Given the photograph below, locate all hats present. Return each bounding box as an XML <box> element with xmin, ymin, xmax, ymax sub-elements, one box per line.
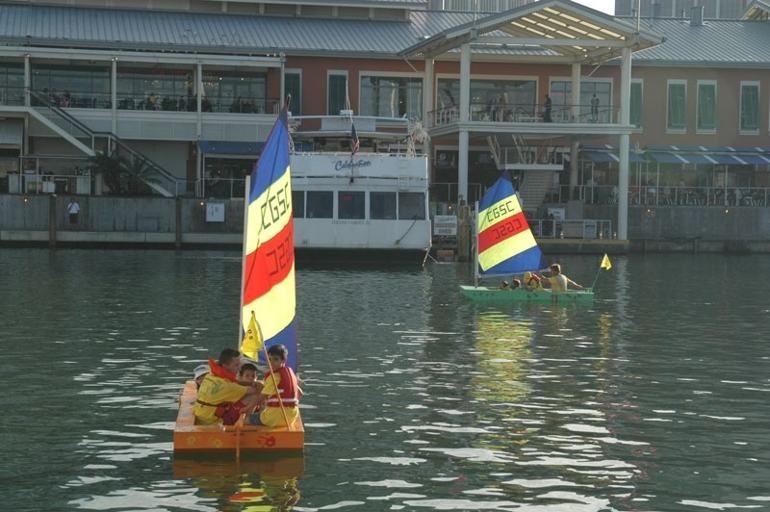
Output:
<box><xmin>193</xmin><ymin>365</ymin><xmax>212</xmax><ymax>380</ymax></box>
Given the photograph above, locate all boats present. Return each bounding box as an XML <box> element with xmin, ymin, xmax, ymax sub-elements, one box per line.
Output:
<box><xmin>283</xmin><ymin>98</ymin><xmax>436</xmax><ymax>270</ymax></box>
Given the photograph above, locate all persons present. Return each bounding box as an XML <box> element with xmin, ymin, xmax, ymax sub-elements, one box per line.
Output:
<box><xmin>29</xmin><ymin>84</ymin><xmax>268</xmax><ymax>115</ymax></box>
<box><xmin>532</xmin><ymin>262</ymin><xmax>585</xmax><ymax>293</ymax></box>
<box><xmin>67</xmin><ymin>196</ymin><xmax>81</xmax><ymax>225</ymax></box>
<box><xmin>589</xmin><ymin>93</ymin><xmax>600</xmax><ymax>122</ymax></box>
<box><xmin>193</xmin><ymin>363</ymin><xmax>210</xmax><ymax>387</ymax></box>
<box><xmin>239</xmin><ymin>362</ymin><xmax>259</xmax><ymax>383</ymax></box>
<box><xmin>585</xmin><ymin>176</ymin><xmax>770</xmax><ymax>208</ymax></box>
<box><xmin>511</xmin><ymin>278</ymin><xmax>523</xmax><ymax>289</ymax></box>
<box><xmin>544</xmin><ymin>93</ymin><xmax>552</xmax><ymax>123</ymax></box>
<box><xmin>239</xmin><ymin>343</ymin><xmax>304</xmax><ymax>428</ymax></box>
<box><xmin>500</xmin><ymin>281</ymin><xmax>509</xmax><ymax>290</ymax></box>
<box><xmin>521</xmin><ymin>271</ymin><xmax>544</xmax><ymax>291</ymax></box>
<box><xmin>192</xmin><ymin>346</ymin><xmax>266</xmax><ymax>426</ymax></box>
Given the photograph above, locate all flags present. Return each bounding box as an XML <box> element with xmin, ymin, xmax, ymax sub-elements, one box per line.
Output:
<box><xmin>599</xmin><ymin>252</ymin><xmax>612</xmax><ymax>271</ymax></box>
<box><xmin>238</xmin><ymin>312</ymin><xmax>263</xmax><ymax>364</ymax></box>
<box><xmin>350</xmin><ymin>122</ymin><xmax>360</xmax><ymax>156</ymax></box>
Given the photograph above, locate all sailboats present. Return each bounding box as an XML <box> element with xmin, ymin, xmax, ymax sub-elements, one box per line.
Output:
<box><xmin>173</xmin><ymin>93</ymin><xmax>314</xmax><ymax>460</ymax></box>
<box><xmin>450</xmin><ymin>166</ymin><xmax>615</xmax><ymax>311</ymax></box>
